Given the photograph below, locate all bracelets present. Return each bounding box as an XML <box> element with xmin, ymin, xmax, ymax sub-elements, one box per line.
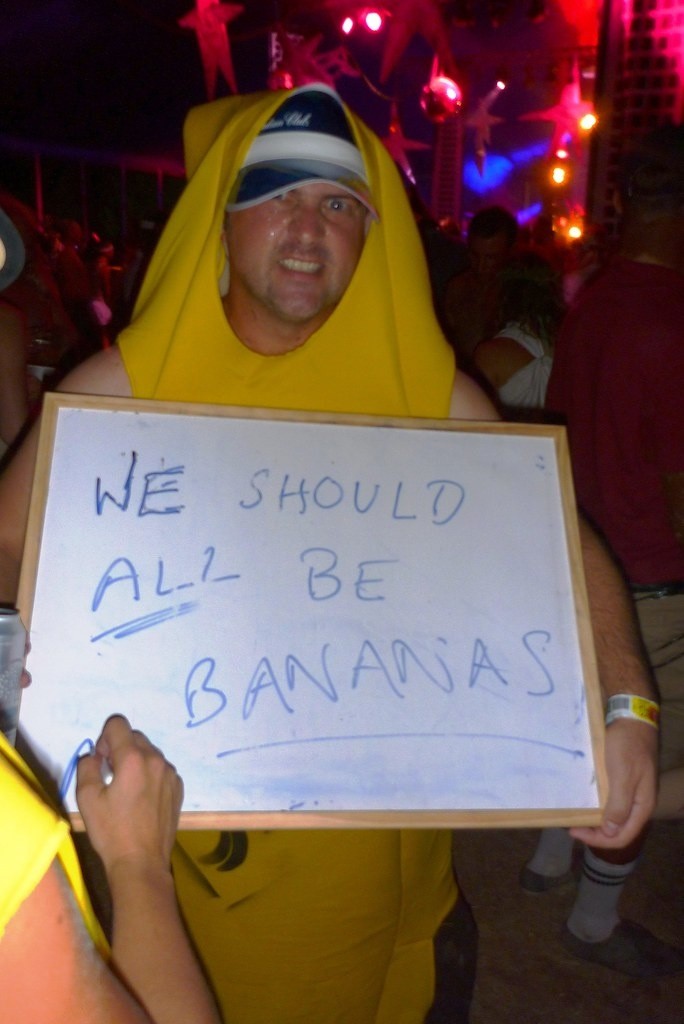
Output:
<box><xmin>604</xmin><ymin>692</ymin><xmax>661</xmax><ymax>728</ymax></box>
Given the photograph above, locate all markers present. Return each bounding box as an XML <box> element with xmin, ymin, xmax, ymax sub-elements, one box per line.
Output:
<box><xmin>89</xmin><ymin>746</ymin><xmax>114</xmax><ymax>785</ymax></box>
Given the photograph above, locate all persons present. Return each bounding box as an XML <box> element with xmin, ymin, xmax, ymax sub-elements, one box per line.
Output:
<box><xmin>1</xmin><ymin>80</ymin><xmax>684</xmax><ymax>1024</ymax></box>
<box><xmin>0</xmin><ymin>632</ymin><xmax>228</xmax><ymax>1024</ymax></box>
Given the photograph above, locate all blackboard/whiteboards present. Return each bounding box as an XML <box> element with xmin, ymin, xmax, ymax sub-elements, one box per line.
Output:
<box><xmin>15</xmin><ymin>393</ymin><xmax>608</xmax><ymax>831</ymax></box>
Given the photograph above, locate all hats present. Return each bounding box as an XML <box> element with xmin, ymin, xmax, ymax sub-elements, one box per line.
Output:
<box><xmin>223</xmin><ymin>83</ymin><xmax>385</xmax><ymax>223</ymax></box>
